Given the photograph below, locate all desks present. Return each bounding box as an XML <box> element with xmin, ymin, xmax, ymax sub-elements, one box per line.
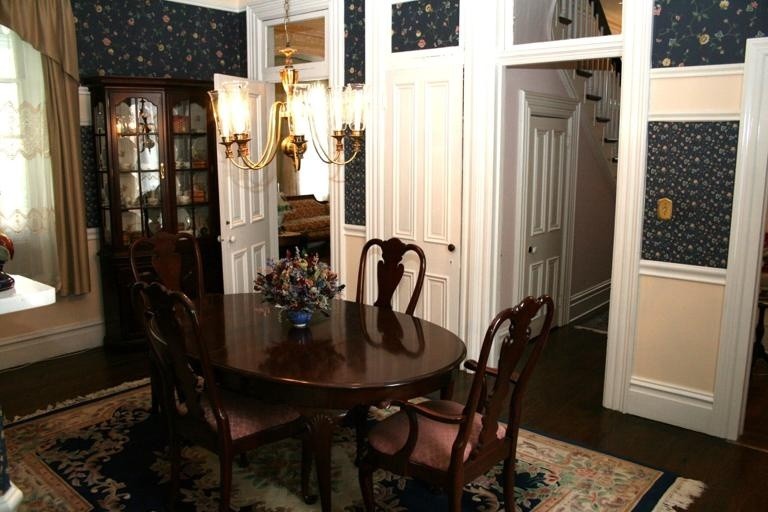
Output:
<box><xmin>279</xmin><ymin>232</ymin><xmax>301</xmax><ymax>258</ymax></box>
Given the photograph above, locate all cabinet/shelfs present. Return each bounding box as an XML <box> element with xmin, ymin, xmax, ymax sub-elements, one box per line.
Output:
<box><xmin>94</xmin><ymin>77</ymin><xmax>221</xmax><ymax>353</ymax></box>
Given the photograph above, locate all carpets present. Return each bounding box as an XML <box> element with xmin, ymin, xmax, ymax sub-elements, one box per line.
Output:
<box><xmin>1</xmin><ymin>377</ymin><xmax>707</xmax><ymax>512</ymax></box>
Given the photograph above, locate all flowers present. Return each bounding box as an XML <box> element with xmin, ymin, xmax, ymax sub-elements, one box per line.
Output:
<box><xmin>253</xmin><ymin>248</ymin><xmax>345</xmax><ymax>322</ymax></box>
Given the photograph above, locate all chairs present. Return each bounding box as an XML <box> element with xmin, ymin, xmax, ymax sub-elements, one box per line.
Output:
<box><xmin>354</xmin><ymin>238</ymin><xmax>426</xmax><ymax>316</ymax></box>
<box><xmin>359</xmin><ymin>295</ymin><xmax>554</xmax><ymax>511</ymax></box>
<box><xmin>128</xmin><ymin>231</ymin><xmax>205</xmax><ymax>298</ymax></box>
<box><xmin>132</xmin><ymin>275</ymin><xmax>335</xmax><ymax>512</ymax></box>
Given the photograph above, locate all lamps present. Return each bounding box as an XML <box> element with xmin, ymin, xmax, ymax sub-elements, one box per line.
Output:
<box><xmin>208</xmin><ymin>1</ymin><xmax>366</xmax><ymax>170</ymax></box>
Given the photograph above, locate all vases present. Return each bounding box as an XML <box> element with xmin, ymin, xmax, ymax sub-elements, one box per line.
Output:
<box><xmin>288</xmin><ymin>310</ymin><xmax>313</xmax><ymax>328</ymax></box>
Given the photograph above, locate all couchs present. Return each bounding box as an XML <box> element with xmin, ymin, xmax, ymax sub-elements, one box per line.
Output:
<box><xmin>278</xmin><ymin>195</ymin><xmax>330</xmax><ymax>239</ymax></box>
